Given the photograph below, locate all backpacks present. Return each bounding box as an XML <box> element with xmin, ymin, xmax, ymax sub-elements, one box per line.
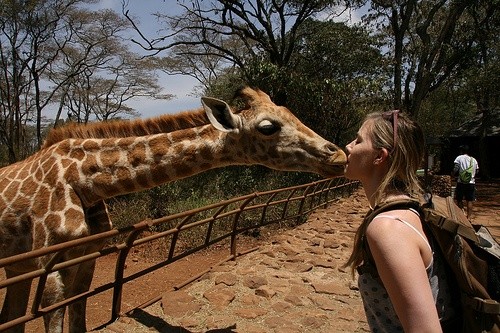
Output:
<box><xmin>356</xmin><ymin>187</ymin><xmax>499</xmax><ymax>332</ymax></box>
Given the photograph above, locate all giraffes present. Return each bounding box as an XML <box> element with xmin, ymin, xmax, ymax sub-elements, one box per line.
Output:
<box><xmin>0</xmin><ymin>86</ymin><xmax>347</xmax><ymax>333</ymax></box>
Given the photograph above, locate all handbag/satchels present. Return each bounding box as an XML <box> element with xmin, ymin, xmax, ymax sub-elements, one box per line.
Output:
<box><xmin>459</xmin><ymin>160</ymin><xmax>473</xmax><ymax>184</ymax></box>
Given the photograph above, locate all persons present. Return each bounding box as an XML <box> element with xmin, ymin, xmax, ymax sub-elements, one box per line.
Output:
<box><xmin>450</xmin><ymin>144</ymin><xmax>480</xmax><ymax>223</ymax></box>
<box><xmin>342</xmin><ymin>110</ymin><xmax>454</xmax><ymax>332</ymax></box>
